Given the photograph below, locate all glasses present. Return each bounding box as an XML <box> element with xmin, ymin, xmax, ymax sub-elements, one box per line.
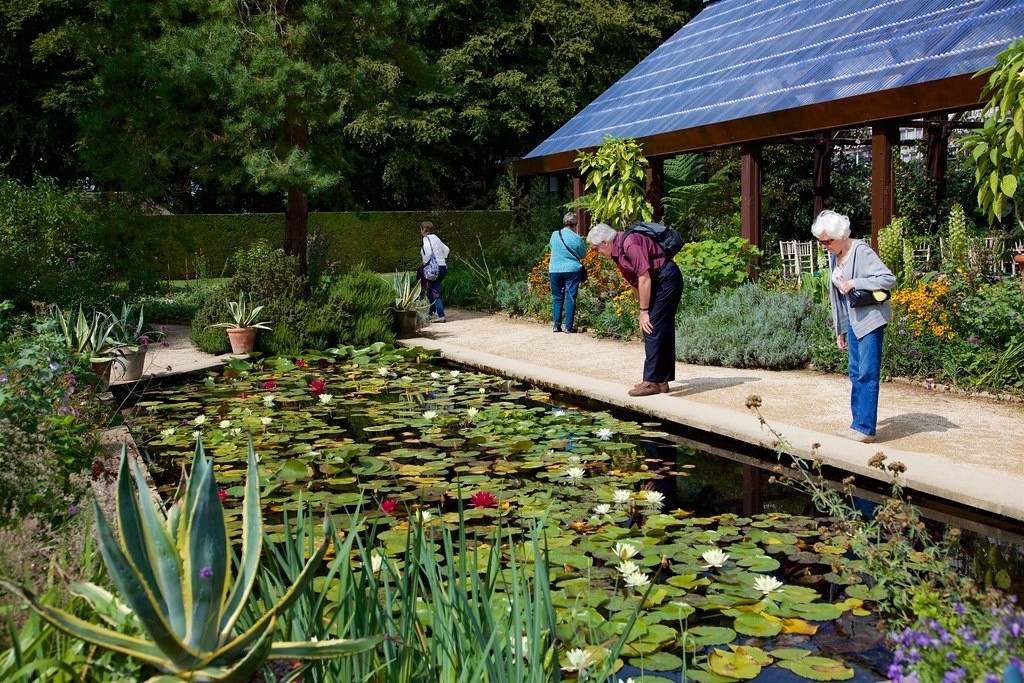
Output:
<box><xmin>819</xmin><ymin>239</ymin><xmax>835</xmax><ymax>245</ymax></box>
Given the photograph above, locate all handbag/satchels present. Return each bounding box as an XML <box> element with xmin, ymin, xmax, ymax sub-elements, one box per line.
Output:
<box><xmin>849</xmin><ymin>244</ymin><xmax>891</xmax><ymax>307</ymax></box>
<box><xmin>423</xmin><ymin>254</ymin><xmax>440</xmax><ymax>281</ymax></box>
<box><xmin>579</xmin><ymin>265</ymin><xmax>588</xmax><ymax>282</ymax></box>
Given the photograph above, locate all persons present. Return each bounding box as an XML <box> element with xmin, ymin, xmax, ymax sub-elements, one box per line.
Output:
<box><xmin>586</xmin><ymin>223</ymin><xmax>683</xmax><ymax>396</ymax></box>
<box><xmin>549</xmin><ymin>212</ymin><xmax>587</xmax><ymax>334</ymax></box>
<box><xmin>420</xmin><ymin>221</ymin><xmax>450</xmax><ymax>323</ymax></box>
<box><xmin>810</xmin><ymin>209</ymin><xmax>897</xmax><ymax>443</ymax></box>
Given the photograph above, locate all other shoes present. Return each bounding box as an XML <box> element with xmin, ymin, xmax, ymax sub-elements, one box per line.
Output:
<box><xmin>553</xmin><ymin>327</ymin><xmax>562</xmax><ymax>331</ymax></box>
<box><xmin>564</xmin><ymin>329</ymin><xmax>574</xmax><ymax>333</ymax></box>
<box><xmin>635</xmin><ymin>382</ymin><xmax>669</xmax><ymax>393</ymax></box>
<box><xmin>629</xmin><ymin>381</ymin><xmax>660</xmax><ymax>396</ymax></box>
<box><xmin>838</xmin><ymin>429</ymin><xmax>874</xmax><ymax>443</ymax></box>
<box><xmin>433</xmin><ymin>317</ymin><xmax>446</xmax><ymax>322</ymax></box>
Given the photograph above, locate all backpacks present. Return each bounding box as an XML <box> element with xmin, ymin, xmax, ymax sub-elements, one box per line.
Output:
<box><xmin>620</xmin><ymin>221</ymin><xmax>687</xmax><ymax>262</ymax></box>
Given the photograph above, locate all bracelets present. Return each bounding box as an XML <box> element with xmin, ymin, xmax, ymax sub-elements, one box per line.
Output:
<box><xmin>638</xmin><ymin>307</ymin><xmax>649</xmax><ymax>311</ymax></box>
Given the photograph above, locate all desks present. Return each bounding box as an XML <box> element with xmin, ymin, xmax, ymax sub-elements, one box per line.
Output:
<box><xmin>1014</xmin><ymin>254</ymin><xmax>1024</xmax><ymax>284</ymax></box>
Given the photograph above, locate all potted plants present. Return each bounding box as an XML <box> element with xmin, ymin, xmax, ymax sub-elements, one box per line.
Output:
<box><xmin>44</xmin><ymin>302</ymin><xmax>139</xmax><ymax>396</ymax></box>
<box><xmin>95</xmin><ymin>302</ymin><xmax>168</xmax><ymax>381</ymax></box>
<box><xmin>379</xmin><ymin>268</ymin><xmax>438</xmax><ymax>336</ymax></box>
<box><xmin>204</xmin><ymin>291</ymin><xmax>274</xmax><ymax>354</ymax></box>
